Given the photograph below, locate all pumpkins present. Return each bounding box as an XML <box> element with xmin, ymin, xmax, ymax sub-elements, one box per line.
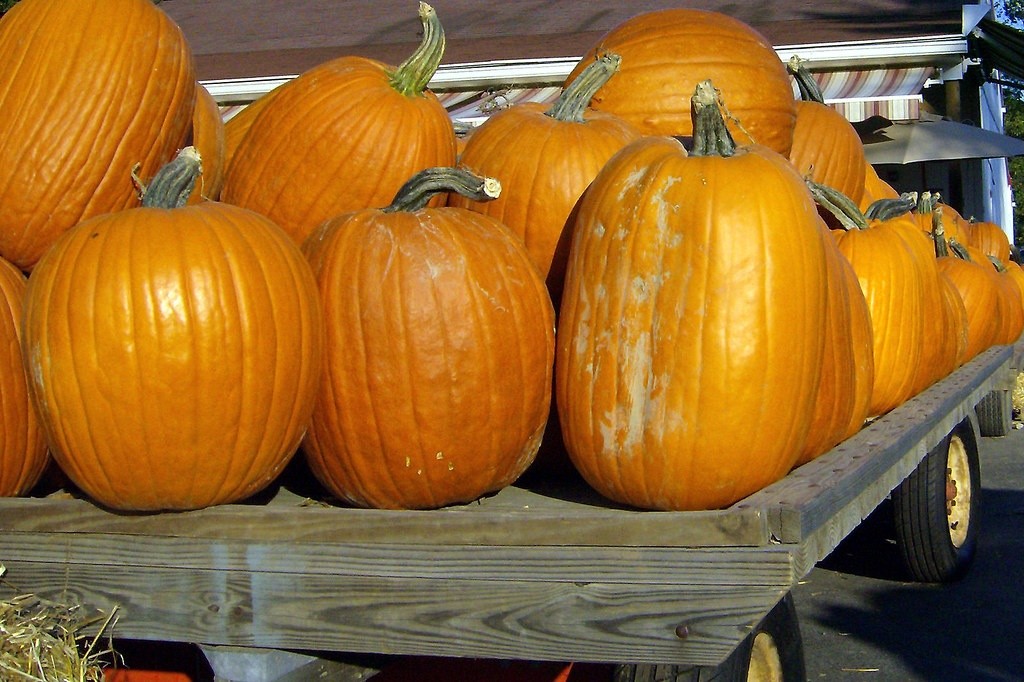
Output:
<box><xmin>0</xmin><ymin>0</ymin><xmax>1024</xmax><ymax>512</ymax></box>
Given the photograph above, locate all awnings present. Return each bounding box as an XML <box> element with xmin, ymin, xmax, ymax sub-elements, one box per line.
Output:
<box><xmin>216</xmin><ymin>62</ymin><xmax>941</xmax><ymax>128</ymax></box>
<box><xmin>967</xmin><ymin>11</ymin><xmax>1024</xmax><ymax>96</ymax></box>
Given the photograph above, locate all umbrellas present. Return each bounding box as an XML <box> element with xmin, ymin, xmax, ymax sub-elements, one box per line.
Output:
<box><xmin>860</xmin><ymin>115</ymin><xmax>1024</xmax><ymax>196</ymax></box>
